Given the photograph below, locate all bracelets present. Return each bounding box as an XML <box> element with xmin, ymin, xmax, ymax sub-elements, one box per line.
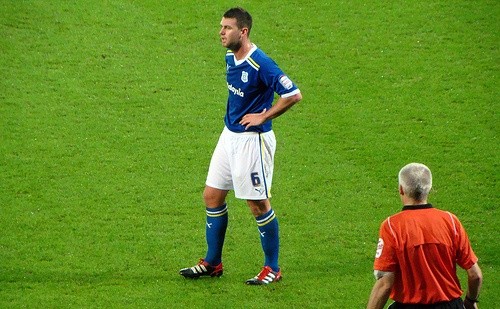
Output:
<box><xmin>465</xmin><ymin>296</ymin><xmax>479</xmax><ymax>303</ymax></box>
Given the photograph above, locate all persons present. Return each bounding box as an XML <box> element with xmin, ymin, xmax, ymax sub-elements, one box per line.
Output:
<box><xmin>365</xmin><ymin>163</ymin><xmax>482</xmax><ymax>309</ymax></box>
<box><xmin>179</xmin><ymin>7</ymin><xmax>303</xmax><ymax>285</ymax></box>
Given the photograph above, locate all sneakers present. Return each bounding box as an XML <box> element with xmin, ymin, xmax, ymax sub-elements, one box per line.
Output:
<box><xmin>245</xmin><ymin>265</ymin><xmax>282</xmax><ymax>286</ymax></box>
<box><xmin>179</xmin><ymin>258</ymin><xmax>223</xmax><ymax>280</ymax></box>
